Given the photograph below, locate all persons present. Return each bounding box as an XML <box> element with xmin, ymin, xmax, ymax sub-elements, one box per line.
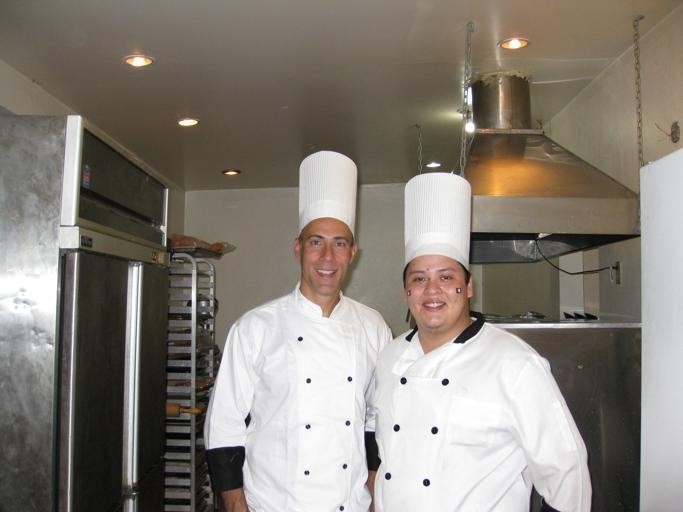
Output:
<box><xmin>361</xmin><ymin>244</ymin><xmax>591</xmax><ymax>512</ymax></box>
<box><xmin>204</xmin><ymin>218</ymin><xmax>397</xmax><ymax>512</ymax></box>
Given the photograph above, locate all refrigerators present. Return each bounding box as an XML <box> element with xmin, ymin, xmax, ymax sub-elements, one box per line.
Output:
<box><xmin>0</xmin><ymin>113</ymin><xmax>170</xmax><ymax>510</ymax></box>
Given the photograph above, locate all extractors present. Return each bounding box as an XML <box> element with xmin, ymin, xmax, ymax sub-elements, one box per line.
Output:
<box><xmin>457</xmin><ymin>75</ymin><xmax>640</xmax><ymax>263</ymax></box>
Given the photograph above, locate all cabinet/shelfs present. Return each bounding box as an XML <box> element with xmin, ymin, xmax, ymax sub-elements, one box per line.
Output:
<box><xmin>164</xmin><ymin>245</ymin><xmax>219</xmax><ymax>512</ymax></box>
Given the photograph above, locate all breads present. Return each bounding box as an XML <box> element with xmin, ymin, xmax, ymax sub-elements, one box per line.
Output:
<box><xmin>171</xmin><ymin>232</ymin><xmax>225</xmax><ymax>254</ymax></box>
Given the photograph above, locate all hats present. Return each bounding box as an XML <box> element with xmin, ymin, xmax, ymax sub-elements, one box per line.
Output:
<box><xmin>298</xmin><ymin>150</ymin><xmax>358</xmax><ymax>240</ymax></box>
<box><xmin>404</xmin><ymin>172</ymin><xmax>472</xmax><ymax>273</ymax></box>
